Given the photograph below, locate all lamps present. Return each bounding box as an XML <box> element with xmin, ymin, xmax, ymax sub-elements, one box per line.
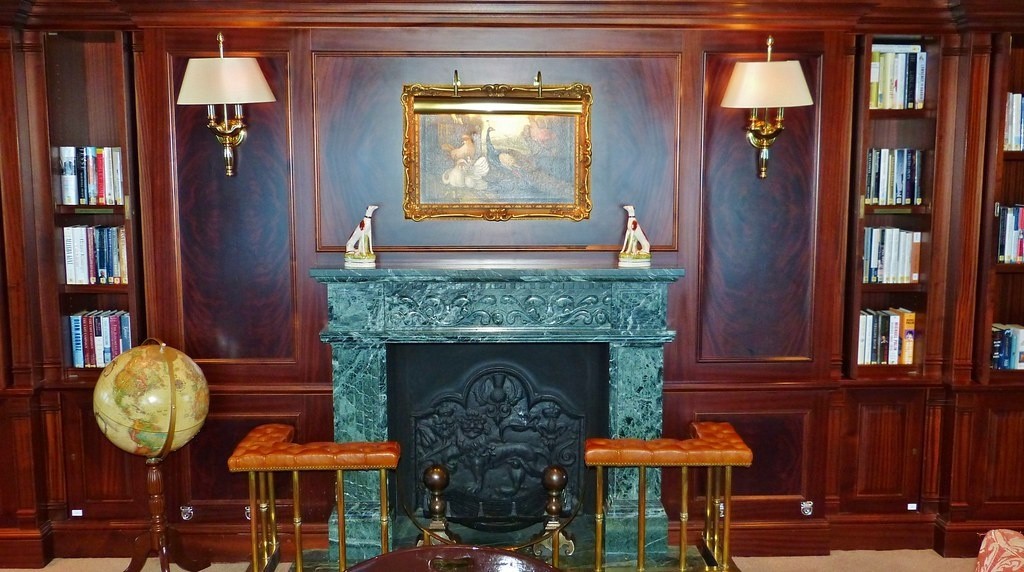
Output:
<box><xmin>721</xmin><ymin>35</ymin><xmax>814</xmax><ymax>181</ymax></box>
<box><xmin>179</xmin><ymin>33</ymin><xmax>276</xmax><ymax>179</ymax></box>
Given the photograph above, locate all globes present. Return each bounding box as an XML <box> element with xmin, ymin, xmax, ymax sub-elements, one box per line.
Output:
<box><xmin>94</xmin><ymin>338</ymin><xmax>211</xmax><ymax>572</ymax></box>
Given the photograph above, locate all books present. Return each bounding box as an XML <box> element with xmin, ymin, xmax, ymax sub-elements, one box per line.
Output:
<box><xmin>64</xmin><ymin>225</ymin><xmax>128</xmax><ymax>285</ymax></box>
<box><xmin>869</xmin><ymin>44</ymin><xmax>926</xmax><ymax>110</ymax></box>
<box><xmin>1003</xmin><ymin>92</ymin><xmax>1024</xmax><ymax>152</ymax></box>
<box><xmin>858</xmin><ymin>307</ymin><xmax>915</xmax><ymax>365</ymax></box>
<box><xmin>69</xmin><ymin>309</ymin><xmax>131</xmax><ymax>369</ymax></box>
<box><xmin>991</xmin><ymin>324</ymin><xmax>1024</xmax><ymax>369</ymax></box>
<box><xmin>59</xmin><ymin>147</ymin><xmax>125</xmax><ymax>206</ymax></box>
<box><xmin>997</xmin><ymin>204</ymin><xmax>1024</xmax><ymax>264</ymax></box>
<box><xmin>865</xmin><ymin>147</ymin><xmax>923</xmax><ymax>206</ymax></box>
<box><xmin>862</xmin><ymin>226</ymin><xmax>922</xmax><ymax>283</ymax></box>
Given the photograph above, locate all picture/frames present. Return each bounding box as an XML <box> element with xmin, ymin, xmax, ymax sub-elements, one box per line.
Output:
<box><xmin>402</xmin><ymin>84</ymin><xmax>592</xmax><ymax>223</ymax></box>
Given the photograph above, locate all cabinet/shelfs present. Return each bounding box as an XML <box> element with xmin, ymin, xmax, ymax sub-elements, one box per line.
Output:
<box><xmin>0</xmin><ymin>22</ymin><xmax>153</xmax><ymax>520</ymax></box>
<box><xmin>975</xmin><ymin>26</ymin><xmax>1024</xmax><ymax>385</ymax></box>
<box><xmin>850</xmin><ymin>30</ymin><xmax>965</xmax><ymax>380</ymax></box>
